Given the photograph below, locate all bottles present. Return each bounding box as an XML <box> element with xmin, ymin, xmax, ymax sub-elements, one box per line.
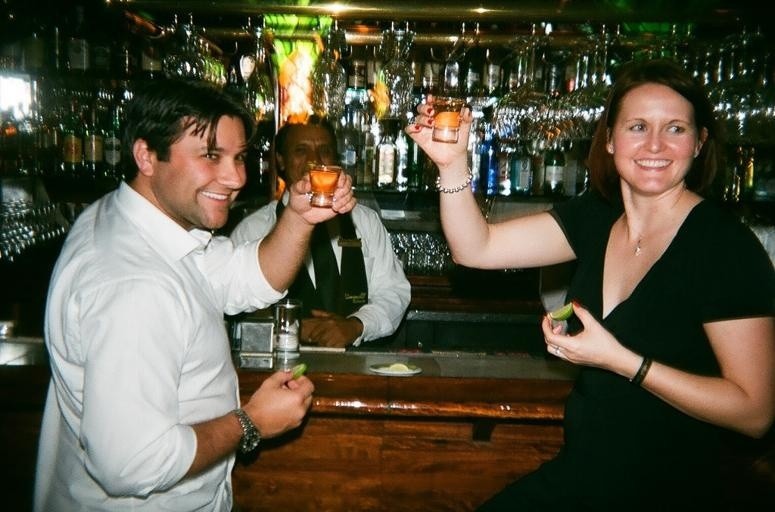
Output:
<box><xmin>723</xmin><ymin>129</ymin><xmax>755</xmax><ymax>203</ymax></box>
<box><xmin>345</xmin><ymin>20</ymin><xmax>775</xmax><ymax>94</ymax></box>
<box><xmin>225</xmin><ymin>39</ymin><xmax>244</xmax><ymax>88</ymax></box>
<box><xmin>342</xmin><ymin>104</ymin><xmax>435</xmax><ymax>194</ymax></box>
<box><xmin>0</xmin><ymin>1</ymin><xmax>160</xmax><ymax>179</ymax></box>
<box><xmin>470</xmin><ymin>99</ymin><xmax>588</xmax><ymax>198</ymax></box>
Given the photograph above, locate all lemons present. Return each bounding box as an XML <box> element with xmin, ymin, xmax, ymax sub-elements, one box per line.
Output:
<box><xmin>289</xmin><ymin>364</ymin><xmax>308</xmax><ymax>378</ymax></box>
<box><xmin>371</xmin><ymin>361</ymin><xmax>416</xmax><ymax>372</ymax></box>
<box><xmin>549</xmin><ymin>303</ymin><xmax>572</xmax><ymax>321</ymax></box>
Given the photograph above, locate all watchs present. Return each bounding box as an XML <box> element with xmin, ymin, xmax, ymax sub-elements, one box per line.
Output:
<box><xmin>232</xmin><ymin>409</ymin><xmax>261</xmax><ymax>454</ymax></box>
<box><xmin>628</xmin><ymin>355</ymin><xmax>653</xmax><ymax>385</ymax></box>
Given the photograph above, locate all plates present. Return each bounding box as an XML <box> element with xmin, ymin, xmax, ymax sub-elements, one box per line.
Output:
<box><xmin>370</xmin><ymin>364</ymin><xmax>422</xmax><ymax>378</ymax></box>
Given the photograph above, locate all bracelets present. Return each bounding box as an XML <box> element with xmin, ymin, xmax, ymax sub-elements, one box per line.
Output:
<box><xmin>436</xmin><ymin>168</ymin><xmax>473</xmax><ymax>193</ymax></box>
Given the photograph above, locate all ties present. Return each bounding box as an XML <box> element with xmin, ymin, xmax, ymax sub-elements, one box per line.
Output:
<box><xmin>312</xmin><ymin>221</ymin><xmax>341</xmax><ymax>314</ymax></box>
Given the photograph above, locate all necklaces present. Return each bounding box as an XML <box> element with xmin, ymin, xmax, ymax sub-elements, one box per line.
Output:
<box><xmin>635</xmin><ymin>234</ymin><xmax>645</xmax><ymax>256</ymax></box>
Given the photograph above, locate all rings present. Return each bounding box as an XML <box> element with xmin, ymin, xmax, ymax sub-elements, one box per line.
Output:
<box><xmin>556</xmin><ymin>349</ymin><xmax>559</xmax><ymax>354</ymax></box>
<box><xmin>556</xmin><ymin>346</ymin><xmax>561</xmax><ymax>350</ymax></box>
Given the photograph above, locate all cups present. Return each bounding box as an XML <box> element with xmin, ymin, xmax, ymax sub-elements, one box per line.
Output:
<box><xmin>272</xmin><ymin>298</ymin><xmax>302</xmax><ymax>352</ymax></box>
<box><xmin>389</xmin><ymin>227</ymin><xmax>451</xmax><ymax>275</ymax></box>
<box><xmin>433</xmin><ymin>93</ymin><xmax>463</xmax><ymax>144</ymax></box>
<box><xmin>273</xmin><ymin>353</ymin><xmax>301</xmax><ymax>373</ymax></box>
<box><xmin>308</xmin><ymin>161</ymin><xmax>341</xmax><ymax>209</ymax></box>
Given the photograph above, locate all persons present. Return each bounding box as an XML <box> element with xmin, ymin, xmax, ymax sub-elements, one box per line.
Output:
<box><xmin>228</xmin><ymin>116</ymin><xmax>411</xmax><ymax>349</ymax></box>
<box><xmin>35</xmin><ymin>80</ymin><xmax>356</xmax><ymax>512</ymax></box>
<box><xmin>406</xmin><ymin>59</ymin><xmax>775</xmax><ymax>512</ymax></box>
